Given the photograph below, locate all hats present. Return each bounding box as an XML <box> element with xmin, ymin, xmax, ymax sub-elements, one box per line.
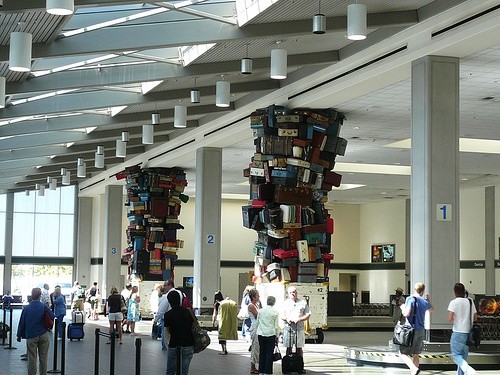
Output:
<box><xmin>395</xmin><ymin>288</ymin><xmax>403</xmax><ymax>293</ymax></box>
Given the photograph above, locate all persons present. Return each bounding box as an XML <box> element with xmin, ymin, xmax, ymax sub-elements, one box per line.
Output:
<box><xmin>447</xmin><ymin>283</ymin><xmax>478</xmax><ymax>375</ymax></box>
<box><xmin>248</xmin><ymin>288</ymin><xmax>260</xmax><ymax>375</ymax></box>
<box><xmin>41</xmin><ymin>284</ymin><xmax>50</xmax><ymax>309</ymax></box>
<box><xmin>16</xmin><ymin>288</ymin><xmax>55</xmax><ymax>375</ymax></box>
<box><xmin>70</xmin><ymin>281</ymin><xmax>89</xmax><ymax>321</ymax></box>
<box><xmin>280</xmin><ymin>286</ymin><xmax>311</xmax><ymax>373</ymax></box>
<box><xmin>164</xmin><ymin>291</ymin><xmax>198</xmax><ymax>375</ymax></box>
<box><xmin>52</xmin><ymin>287</ymin><xmax>66</xmax><ymax>341</ymax></box>
<box><xmin>106</xmin><ymin>285</ymin><xmax>126</xmax><ymax>345</ymax></box>
<box><xmin>242</xmin><ymin>286</ymin><xmax>255</xmax><ymax>337</ymax></box>
<box><xmin>2</xmin><ymin>290</ymin><xmax>14</xmax><ymax>310</ymax></box>
<box><xmin>150</xmin><ymin>284</ymin><xmax>161</xmax><ymax>324</ymax></box>
<box><xmin>254</xmin><ymin>296</ymin><xmax>279</xmax><ymax>375</ymax></box>
<box><xmin>128</xmin><ymin>285</ymin><xmax>140</xmax><ymax>336</ymax></box>
<box><xmin>47</xmin><ymin>285</ymin><xmax>66</xmax><ymax>332</ymax></box>
<box><xmin>154</xmin><ymin>279</ymin><xmax>185</xmax><ymax>350</ymax></box>
<box><xmin>120</xmin><ymin>283</ymin><xmax>132</xmax><ymax>334</ymax></box>
<box><xmin>399</xmin><ymin>282</ymin><xmax>433</xmax><ymax>375</ymax></box>
<box><xmin>390</xmin><ymin>287</ymin><xmax>406</xmax><ymax>342</ymax></box>
<box><xmin>213</xmin><ymin>291</ymin><xmax>227</xmax><ymax>355</ymax></box>
<box><xmin>85</xmin><ymin>282</ymin><xmax>101</xmax><ymax>320</ymax></box>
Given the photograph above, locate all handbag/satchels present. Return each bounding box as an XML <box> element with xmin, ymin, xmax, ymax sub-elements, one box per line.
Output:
<box><xmin>187</xmin><ymin>306</ymin><xmax>210</xmax><ymax>354</ymax></box>
<box><xmin>120</xmin><ymin>295</ymin><xmax>128</xmax><ymax>313</ymax></box>
<box><xmin>466</xmin><ymin>299</ymin><xmax>483</xmax><ymax>347</ymax></box>
<box><xmin>237</xmin><ymin>296</ymin><xmax>249</xmax><ymax>320</ymax></box>
<box><xmin>182</xmin><ymin>292</ymin><xmax>194</xmax><ymax>314</ymax></box>
<box><xmin>44</xmin><ymin>308</ymin><xmax>53</xmax><ymax>329</ymax></box>
<box><xmin>272</xmin><ymin>345</ymin><xmax>281</xmax><ymax>361</ymax></box>
<box><xmin>393</xmin><ymin>297</ymin><xmax>416</xmax><ymax>347</ymax></box>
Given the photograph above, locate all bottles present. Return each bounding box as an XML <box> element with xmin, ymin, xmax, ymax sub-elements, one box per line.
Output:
<box><xmin>389</xmin><ymin>338</ymin><xmax>393</xmax><ymax>348</ymax></box>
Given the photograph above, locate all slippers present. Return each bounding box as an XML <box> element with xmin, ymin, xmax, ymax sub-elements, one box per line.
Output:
<box><xmin>250</xmin><ymin>370</ymin><xmax>258</xmax><ymax>373</ymax></box>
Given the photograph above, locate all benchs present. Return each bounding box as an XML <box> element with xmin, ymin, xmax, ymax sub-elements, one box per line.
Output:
<box><xmin>27</xmin><ymin>296</ymin><xmax>49</xmax><ymax>307</ymax></box>
<box><xmin>0</xmin><ymin>295</ymin><xmax>23</xmax><ymax>309</ymax></box>
<box><xmin>61</xmin><ymin>294</ymin><xmax>72</xmax><ymax>308</ymax></box>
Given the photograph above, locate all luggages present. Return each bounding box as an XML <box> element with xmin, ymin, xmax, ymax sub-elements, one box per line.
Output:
<box><xmin>72</xmin><ymin>306</ymin><xmax>85</xmax><ymax>323</ymax></box>
<box><xmin>68</xmin><ymin>311</ymin><xmax>85</xmax><ymax>342</ymax></box>
<box><xmin>241</xmin><ymin>104</ymin><xmax>348</xmax><ymax>284</ymax></box>
<box><xmin>124</xmin><ymin>165</ymin><xmax>189</xmax><ymax>281</ymax></box>
<box><xmin>282</xmin><ymin>321</ymin><xmax>304</xmax><ymax>374</ymax></box>
